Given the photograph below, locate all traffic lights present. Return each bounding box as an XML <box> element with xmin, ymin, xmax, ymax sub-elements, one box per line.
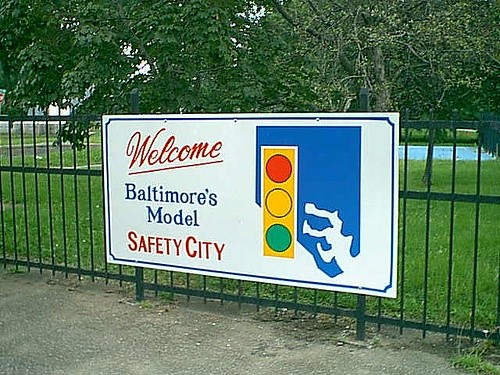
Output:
<box><xmin>261</xmin><ymin>145</ymin><xmax>297</xmax><ymax>261</ymax></box>
